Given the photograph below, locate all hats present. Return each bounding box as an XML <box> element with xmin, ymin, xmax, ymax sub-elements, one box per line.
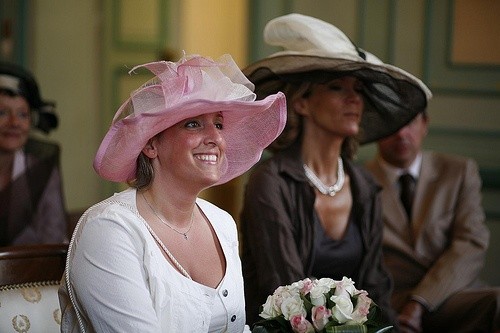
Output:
<box><xmin>240</xmin><ymin>11</ymin><xmax>433</xmax><ymax>152</ymax></box>
<box><xmin>92</xmin><ymin>50</ymin><xmax>287</xmax><ymax>186</ymax></box>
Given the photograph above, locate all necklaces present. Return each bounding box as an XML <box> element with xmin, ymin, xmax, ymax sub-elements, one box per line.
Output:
<box><xmin>300</xmin><ymin>156</ymin><xmax>346</xmax><ymax>197</ymax></box>
<box><xmin>140</xmin><ymin>188</ymin><xmax>195</xmax><ymax>239</ymax></box>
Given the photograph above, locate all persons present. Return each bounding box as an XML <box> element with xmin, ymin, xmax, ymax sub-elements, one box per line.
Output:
<box><xmin>361</xmin><ymin>104</ymin><xmax>490</xmax><ymax>333</ymax></box>
<box><xmin>58</xmin><ymin>51</ymin><xmax>287</xmax><ymax>333</ymax></box>
<box><xmin>238</xmin><ymin>13</ymin><xmax>432</xmax><ymax>333</ymax></box>
<box><xmin>0</xmin><ymin>16</ymin><xmax>60</xmax><ymax>136</ymax></box>
<box><xmin>0</xmin><ymin>92</ymin><xmax>68</xmax><ymax>245</ymax></box>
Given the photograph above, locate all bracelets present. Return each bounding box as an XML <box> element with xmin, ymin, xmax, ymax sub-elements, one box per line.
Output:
<box><xmin>408</xmin><ymin>293</ymin><xmax>431</xmax><ymax>312</ymax></box>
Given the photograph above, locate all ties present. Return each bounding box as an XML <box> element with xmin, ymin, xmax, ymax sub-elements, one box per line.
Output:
<box><xmin>397</xmin><ymin>174</ymin><xmax>415</xmax><ymax>225</ymax></box>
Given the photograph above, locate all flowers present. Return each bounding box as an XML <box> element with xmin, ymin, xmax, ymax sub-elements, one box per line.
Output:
<box><xmin>253</xmin><ymin>276</ymin><xmax>399</xmax><ymax>333</ymax></box>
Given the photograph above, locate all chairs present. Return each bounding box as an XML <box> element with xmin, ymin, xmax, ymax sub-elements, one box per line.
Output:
<box><xmin>0</xmin><ymin>243</ymin><xmax>69</xmax><ymax>333</ymax></box>
